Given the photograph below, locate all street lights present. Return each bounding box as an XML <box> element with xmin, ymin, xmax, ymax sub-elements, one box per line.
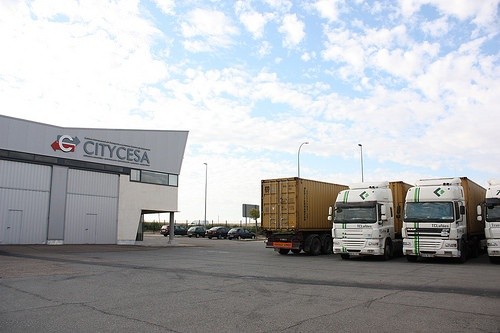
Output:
<box><xmin>203</xmin><ymin>163</ymin><xmax>207</xmax><ymax>225</ymax></box>
<box><xmin>358</xmin><ymin>144</ymin><xmax>363</xmax><ymax>182</ymax></box>
<box><xmin>298</xmin><ymin>142</ymin><xmax>309</xmax><ymax>177</ymax></box>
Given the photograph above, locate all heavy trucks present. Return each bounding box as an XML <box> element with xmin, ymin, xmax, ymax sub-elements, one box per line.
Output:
<box><xmin>328</xmin><ymin>181</ymin><xmax>414</xmax><ymax>261</ymax></box>
<box><xmin>260</xmin><ymin>177</ymin><xmax>350</xmax><ymax>257</ymax></box>
<box><xmin>397</xmin><ymin>177</ymin><xmax>487</xmax><ymax>261</ymax></box>
<box><xmin>477</xmin><ymin>179</ymin><xmax>500</xmax><ymax>263</ymax></box>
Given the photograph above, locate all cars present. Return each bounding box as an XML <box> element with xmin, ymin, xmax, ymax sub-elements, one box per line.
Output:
<box><xmin>187</xmin><ymin>226</ymin><xmax>206</xmax><ymax>238</ymax></box>
<box><xmin>206</xmin><ymin>227</ymin><xmax>227</xmax><ymax>239</ymax></box>
<box><xmin>160</xmin><ymin>225</ymin><xmax>187</xmax><ymax>237</ymax></box>
<box><xmin>227</xmin><ymin>227</ymin><xmax>256</xmax><ymax>240</ymax></box>
